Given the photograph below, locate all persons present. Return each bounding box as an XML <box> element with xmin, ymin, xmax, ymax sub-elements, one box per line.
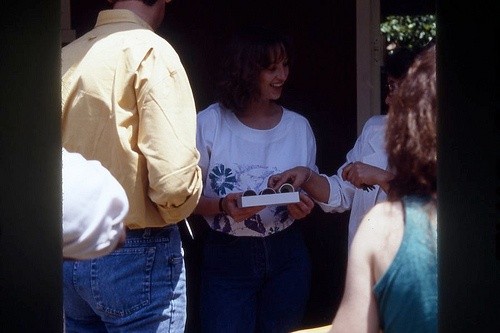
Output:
<box><xmin>191</xmin><ymin>20</ymin><xmax>318</xmax><ymax>333</ymax></box>
<box><xmin>329</xmin><ymin>44</ymin><xmax>438</xmax><ymax>333</ymax></box>
<box><xmin>267</xmin><ymin>44</ymin><xmax>429</xmax><ymax>259</ymax></box>
<box><xmin>60</xmin><ymin>0</ymin><xmax>203</xmax><ymax>333</ymax></box>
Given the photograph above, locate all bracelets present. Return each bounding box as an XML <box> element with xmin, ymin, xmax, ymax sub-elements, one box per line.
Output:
<box><xmin>305</xmin><ymin>168</ymin><xmax>313</xmax><ymax>183</ymax></box>
<box><xmin>219</xmin><ymin>197</ymin><xmax>225</xmax><ymax>214</ymax></box>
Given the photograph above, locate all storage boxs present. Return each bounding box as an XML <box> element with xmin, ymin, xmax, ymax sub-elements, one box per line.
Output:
<box><xmin>237</xmin><ymin>191</ymin><xmax>300</xmax><ymax>208</ymax></box>
